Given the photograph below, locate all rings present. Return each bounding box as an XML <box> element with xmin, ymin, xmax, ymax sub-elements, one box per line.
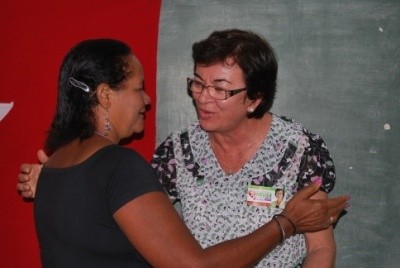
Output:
<box><xmin>331</xmin><ymin>216</ymin><xmax>334</xmax><ymax>224</ymax></box>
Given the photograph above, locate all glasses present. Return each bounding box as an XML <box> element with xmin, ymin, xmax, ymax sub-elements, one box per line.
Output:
<box><xmin>187</xmin><ymin>77</ymin><xmax>249</xmax><ymax>100</ymax></box>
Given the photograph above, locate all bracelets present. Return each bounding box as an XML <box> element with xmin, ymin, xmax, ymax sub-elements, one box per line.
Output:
<box><xmin>272</xmin><ymin>214</ymin><xmax>296</xmax><ymax>243</ymax></box>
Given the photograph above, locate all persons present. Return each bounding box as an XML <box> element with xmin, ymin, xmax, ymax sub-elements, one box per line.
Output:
<box><xmin>272</xmin><ymin>189</ymin><xmax>286</xmax><ymax>209</ymax></box>
<box><xmin>17</xmin><ymin>29</ymin><xmax>337</xmax><ymax>268</ymax></box>
<box><xmin>32</xmin><ymin>37</ymin><xmax>351</xmax><ymax>268</ymax></box>
